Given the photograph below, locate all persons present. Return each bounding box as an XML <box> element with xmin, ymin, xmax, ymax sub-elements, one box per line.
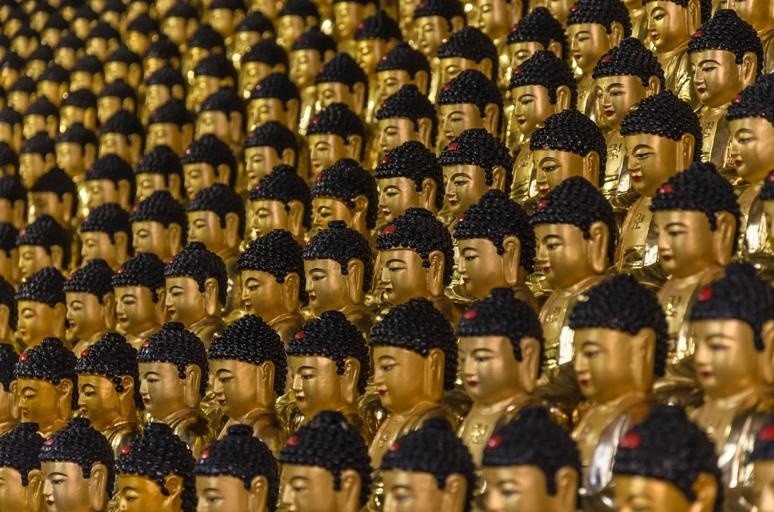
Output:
<box><xmin>2</xmin><ymin>2</ymin><xmax>772</xmax><ymax>512</ymax></box>
